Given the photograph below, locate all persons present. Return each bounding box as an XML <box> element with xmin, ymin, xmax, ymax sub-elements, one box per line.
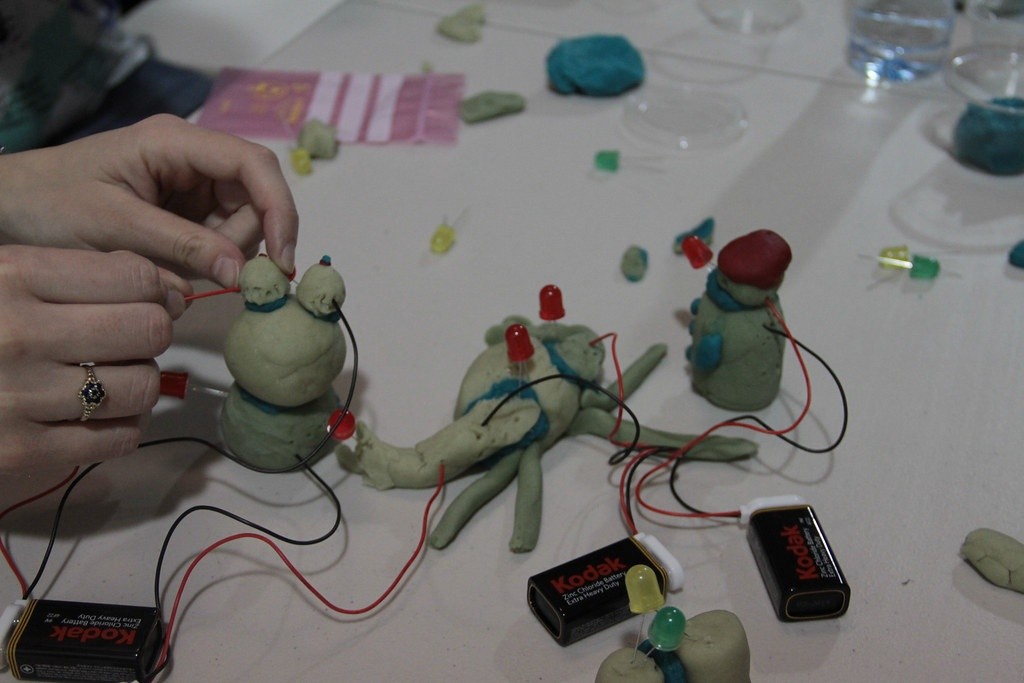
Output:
<box><xmin>0</xmin><ymin>113</ymin><xmax>299</xmax><ymax>473</ymax></box>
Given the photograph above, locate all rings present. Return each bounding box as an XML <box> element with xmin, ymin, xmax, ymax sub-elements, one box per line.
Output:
<box><xmin>76</xmin><ymin>362</ymin><xmax>106</xmax><ymax>424</ymax></box>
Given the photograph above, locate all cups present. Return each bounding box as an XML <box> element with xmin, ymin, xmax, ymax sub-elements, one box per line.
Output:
<box><xmin>945</xmin><ymin>0</ymin><xmax>1024</xmax><ymax>176</ymax></box>
<box><xmin>696</xmin><ymin>0</ymin><xmax>804</xmax><ymax>34</ymax></box>
<box><xmin>842</xmin><ymin>0</ymin><xmax>955</xmax><ymax>82</ymax></box>
